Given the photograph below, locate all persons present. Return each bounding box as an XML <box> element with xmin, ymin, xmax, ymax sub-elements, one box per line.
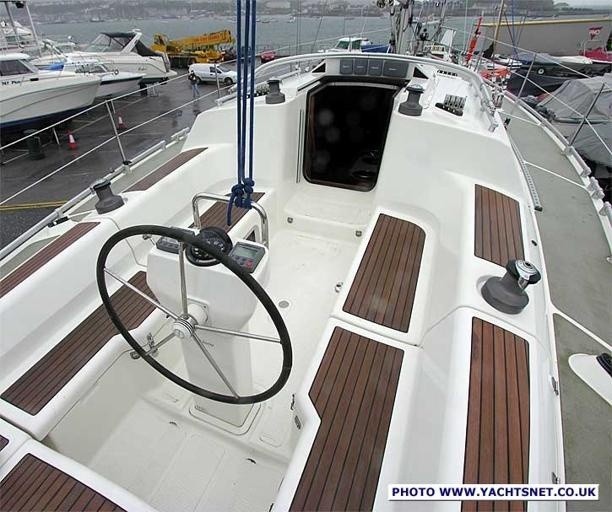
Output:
<box><xmin>187</xmin><ymin>70</ymin><xmax>202</xmax><ymax>99</ymax></box>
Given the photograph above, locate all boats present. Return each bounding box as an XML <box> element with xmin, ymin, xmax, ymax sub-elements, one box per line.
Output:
<box><xmin>0</xmin><ymin>0</ymin><xmax>612</xmax><ymax>511</ymax></box>
